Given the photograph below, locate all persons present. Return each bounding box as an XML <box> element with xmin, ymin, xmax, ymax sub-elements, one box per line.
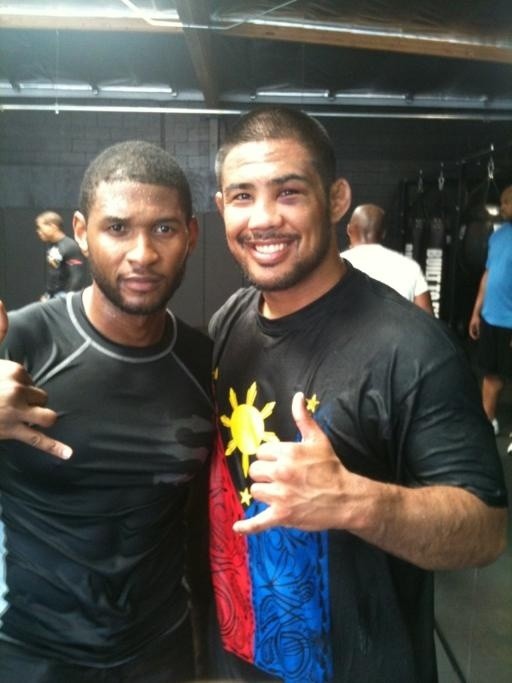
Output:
<box><xmin>209</xmin><ymin>103</ymin><xmax>512</xmax><ymax>682</ymax></box>
<box><xmin>340</xmin><ymin>202</ymin><xmax>433</xmax><ymax>317</ymax></box>
<box><xmin>33</xmin><ymin>210</ymin><xmax>85</xmax><ymax>300</ymax></box>
<box><xmin>0</xmin><ymin>138</ymin><xmax>215</xmax><ymax>683</ymax></box>
<box><xmin>468</xmin><ymin>187</ymin><xmax>512</xmax><ymax>455</ymax></box>
